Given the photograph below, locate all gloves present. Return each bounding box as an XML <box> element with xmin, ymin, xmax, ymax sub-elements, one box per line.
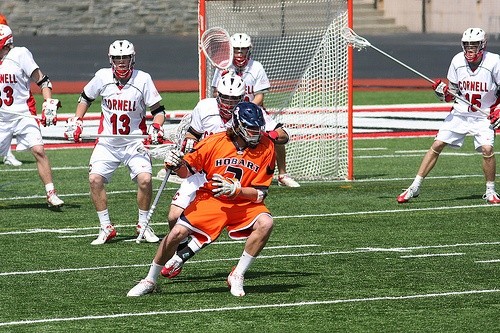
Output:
<box><xmin>149</xmin><ymin>123</ymin><xmax>164</xmax><ymax>145</ymax></box>
<box><xmin>63</xmin><ymin>117</ymin><xmax>83</xmax><ymax>143</ymax></box>
<box><xmin>211</xmin><ymin>173</ymin><xmax>242</xmax><ymax>200</ymax></box>
<box><xmin>487</xmin><ymin>104</ymin><xmax>500</xmax><ymax>129</ymax></box>
<box><xmin>431</xmin><ymin>79</ymin><xmax>449</xmax><ymax>101</ymax></box>
<box><xmin>181</xmin><ymin>137</ymin><xmax>198</xmax><ymax>155</ymax></box>
<box><xmin>42</xmin><ymin>98</ymin><xmax>62</xmax><ymax>127</ymax></box>
<box><xmin>164</xmin><ymin>149</ymin><xmax>184</xmax><ymax>172</ymax></box>
<box><xmin>266</xmin><ymin>130</ymin><xmax>279</xmax><ymax>142</ymax></box>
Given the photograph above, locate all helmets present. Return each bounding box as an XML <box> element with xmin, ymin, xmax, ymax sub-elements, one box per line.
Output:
<box><xmin>0</xmin><ymin>24</ymin><xmax>13</xmax><ymax>51</ymax></box>
<box><xmin>233</xmin><ymin>102</ymin><xmax>266</xmax><ymax>148</ymax></box>
<box><xmin>108</xmin><ymin>40</ymin><xmax>136</xmax><ymax>79</ymax></box>
<box><xmin>218</xmin><ymin>74</ymin><xmax>246</xmax><ymax>119</ymax></box>
<box><xmin>460</xmin><ymin>27</ymin><xmax>487</xmax><ymax>63</ymax></box>
<box><xmin>231</xmin><ymin>32</ymin><xmax>251</xmax><ymax>67</ymax></box>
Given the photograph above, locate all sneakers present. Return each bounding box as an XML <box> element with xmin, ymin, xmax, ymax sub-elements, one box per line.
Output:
<box><xmin>48</xmin><ymin>195</ymin><xmax>64</xmax><ymax>208</ymax></box>
<box><xmin>90</xmin><ymin>225</ymin><xmax>116</xmax><ymax>245</ymax></box>
<box><xmin>278</xmin><ymin>174</ymin><xmax>300</xmax><ymax>187</ymax></box>
<box><xmin>127</xmin><ymin>279</ymin><xmax>159</xmax><ymax>296</ymax></box>
<box><xmin>397</xmin><ymin>186</ymin><xmax>420</xmax><ymax>203</ymax></box>
<box><xmin>137</xmin><ymin>224</ymin><xmax>159</xmax><ymax>243</ymax></box>
<box><xmin>161</xmin><ymin>259</ymin><xmax>179</xmax><ymax>277</ymax></box>
<box><xmin>3</xmin><ymin>156</ymin><xmax>22</xmax><ymax>166</ymax></box>
<box><xmin>168</xmin><ymin>265</ymin><xmax>182</xmax><ymax>278</ymax></box>
<box><xmin>228</xmin><ymin>272</ymin><xmax>245</xmax><ymax>296</ymax></box>
<box><xmin>482</xmin><ymin>190</ymin><xmax>500</xmax><ymax>203</ymax></box>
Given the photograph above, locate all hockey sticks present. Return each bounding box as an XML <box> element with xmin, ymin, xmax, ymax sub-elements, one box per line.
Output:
<box><xmin>201</xmin><ymin>26</ymin><xmax>233</xmax><ymax>70</ymax></box>
<box><xmin>137</xmin><ymin>143</ymin><xmax>192</xmax><ymax>161</ymax></box>
<box><xmin>79</xmin><ymin>133</ymin><xmax>175</xmax><ymax>142</ymax></box>
<box><xmin>0</xmin><ymin>108</ymin><xmax>42</xmax><ymax>120</ymax></box>
<box><xmin>340</xmin><ymin>27</ymin><xmax>489</xmax><ymax>116</ymax></box>
<box><xmin>135</xmin><ymin>112</ymin><xmax>193</xmax><ymax>245</ymax></box>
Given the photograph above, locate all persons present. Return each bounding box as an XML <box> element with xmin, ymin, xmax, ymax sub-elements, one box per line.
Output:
<box><xmin>0</xmin><ymin>24</ymin><xmax>65</xmax><ymax>208</ymax></box>
<box><xmin>397</xmin><ymin>28</ymin><xmax>500</xmax><ymax>205</ymax></box>
<box><xmin>0</xmin><ymin>14</ymin><xmax>22</xmax><ymax>166</ymax></box>
<box><xmin>212</xmin><ymin>33</ymin><xmax>300</xmax><ymax>188</ymax></box>
<box><xmin>160</xmin><ymin>74</ymin><xmax>288</xmax><ymax>279</ymax></box>
<box><xmin>126</xmin><ymin>102</ymin><xmax>276</xmax><ymax>298</ymax></box>
<box><xmin>63</xmin><ymin>40</ymin><xmax>165</xmax><ymax>245</ymax></box>
